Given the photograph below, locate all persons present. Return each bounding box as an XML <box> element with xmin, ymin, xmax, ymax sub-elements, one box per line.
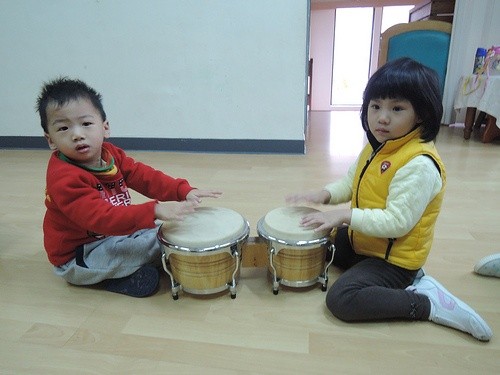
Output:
<box><xmin>36</xmin><ymin>78</ymin><xmax>222</xmax><ymax>297</ymax></box>
<box><xmin>284</xmin><ymin>55</ymin><xmax>493</xmax><ymax>342</ymax></box>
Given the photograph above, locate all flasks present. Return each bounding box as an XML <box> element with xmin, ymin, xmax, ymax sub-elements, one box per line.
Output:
<box><xmin>473</xmin><ymin>48</ymin><xmax>486</xmax><ymax>73</ymax></box>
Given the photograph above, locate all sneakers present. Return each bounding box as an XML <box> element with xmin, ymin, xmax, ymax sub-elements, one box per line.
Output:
<box><xmin>406</xmin><ymin>268</ymin><xmax>492</xmax><ymax>341</ymax></box>
<box><xmin>474</xmin><ymin>253</ymin><xmax>500</xmax><ymax>277</ymax></box>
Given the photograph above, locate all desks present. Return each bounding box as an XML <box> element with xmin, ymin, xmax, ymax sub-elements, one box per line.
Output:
<box><xmin>453</xmin><ymin>72</ymin><xmax>500</xmax><ymax>143</ymax></box>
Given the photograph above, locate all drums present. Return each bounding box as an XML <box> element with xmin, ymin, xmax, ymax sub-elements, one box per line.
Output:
<box><xmin>256</xmin><ymin>205</ymin><xmax>337</xmax><ymax>295</ymax></box>
<box><xmin>156</xmin><ymin>205</ymin><xmax>251</xmax><ymax>301</ymax></box>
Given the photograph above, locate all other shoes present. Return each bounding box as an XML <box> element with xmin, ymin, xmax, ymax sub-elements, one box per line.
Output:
<box><xmin>102</xmin><ymin>266</ymin><xmax>160</xmax><ymax>297</ymax></box>
